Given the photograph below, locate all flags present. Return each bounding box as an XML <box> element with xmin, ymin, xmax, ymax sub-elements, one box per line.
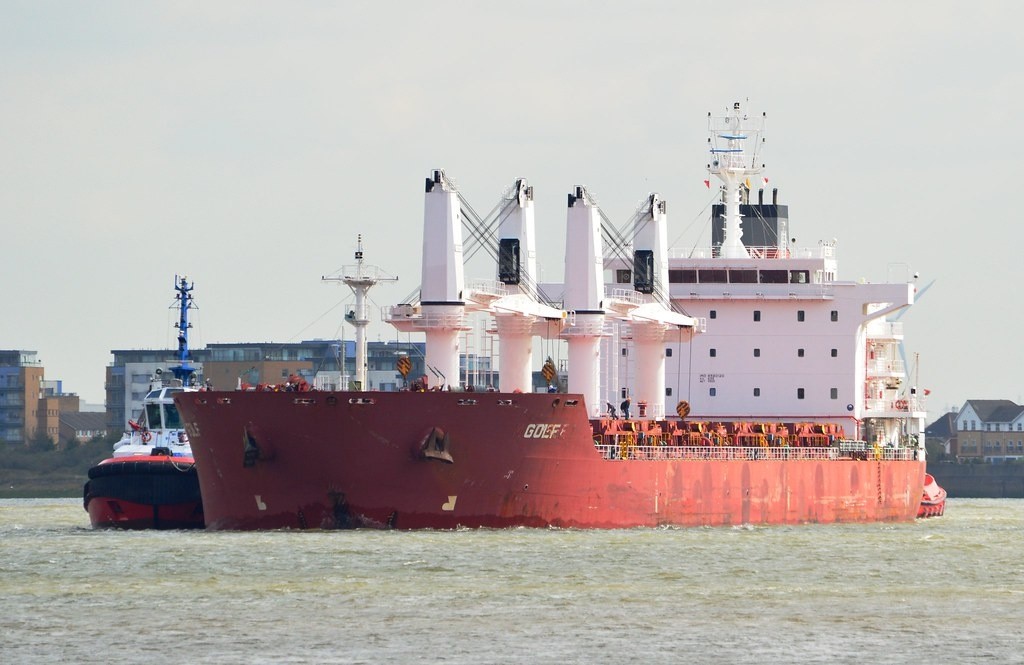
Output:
<box><xmin>745</xmin><ymin>178</ymin><xmax>751</xmax><ymax>188</ymax></box>
<box><xmin>764</xmin><ymin>178</ymin><xmax>768</xmax><ymax>183</ymax></box>
<box><xmin>704</xmin><ymin>180</ymin><xmax>709</xmax><ymax>188</ymax></box>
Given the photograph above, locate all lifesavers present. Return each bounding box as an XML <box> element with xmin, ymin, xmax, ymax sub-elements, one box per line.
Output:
<box><xmin>180</xmin><ymin>433</ymin><xmax>189</xmax><ymax>442</ymax></box>
<box><xmin>156</xmin><ymin>368</ymin><xmax>163</xmax><ymax>375</ymax></box>
<box><xmin>142</xmin><ymin>432</ymin><xmax>152</xmax><ymax>442</ymax></box>
<box><xmin>895</xmin><ymin>400</ymin><xmax>905</xmax><ymax>410</ymax></box>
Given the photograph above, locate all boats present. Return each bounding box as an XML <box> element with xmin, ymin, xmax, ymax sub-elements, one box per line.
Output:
<box><xmin>82</xmin><ymin>274</ymin><xmax>204</xmax><ymax>530</ymax></box>
<box><xmin>174</xmin><ymin>90</ymin><xmax>929</xmax><ymax>531</ymax></box>
<box><xmin>917</xmin><ymin>473</ymin><xmax>947</xmax><ymax>519</ymax></box>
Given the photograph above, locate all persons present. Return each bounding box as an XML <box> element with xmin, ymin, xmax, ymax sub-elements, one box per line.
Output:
<box><xmin>205</xmin><ymin>376</ymin><xmax>212</xmax><ymax>392</ymax></box>
<box><xmin>607</xmin><ymin>403</ymin><xmax>619</xmax><ymax>420</ymax></box>
<box><xmin>415</xmin><ymin>378</ymin><xmax>426</xmax><ymax>390</ymax></box>
<box><xmin>487</xmin><ymin>384</ymin><xmax>495</xmax><ymax>393</ymax></box>
<box><xmin>621</xmin><ymin>398</ymin><xmax>631</xmax><ymax>420</ymax></box>
<box><xmin>286</xmin><ymin>382</ymin><xmax>294</xmax><ymax>392</ymax></box>
